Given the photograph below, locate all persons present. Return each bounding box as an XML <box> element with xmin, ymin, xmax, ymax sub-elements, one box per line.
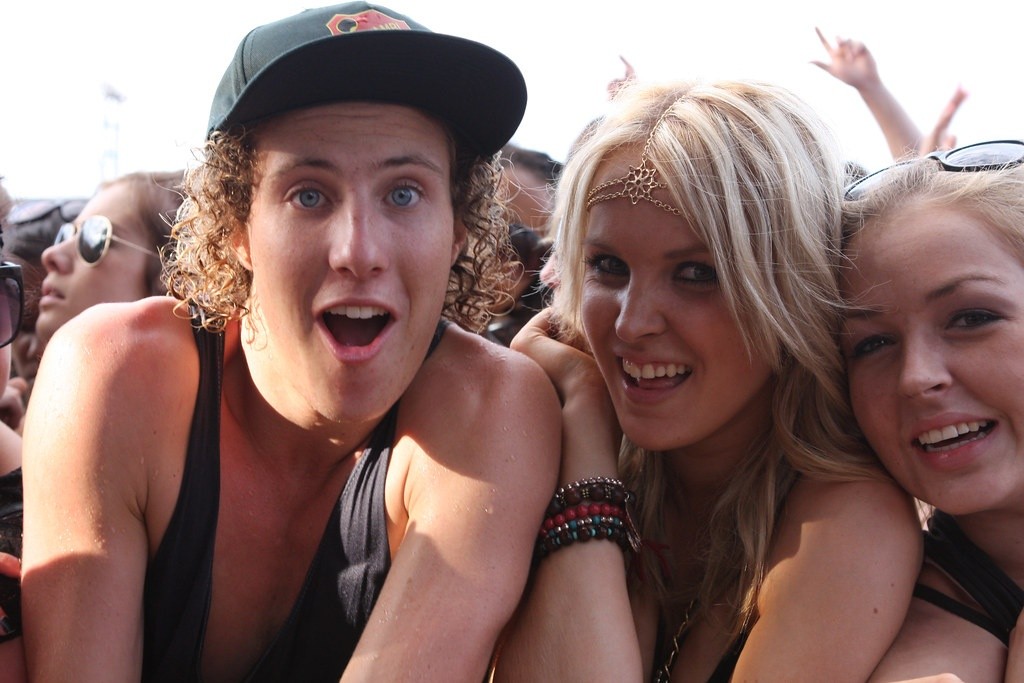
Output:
<box><xmin>22</xmin><ymin>1</ymin><xmax>566</xmax><ymax>683</ymax></box>
<box><xmin>0</xmin><ymin>27</ymin><xmax>1024</xmax><ymax>683</ymax></box>
<box><xmin>489</xmin><ymin>75</ymin><xmax>922</xmax><ymax>683</ymax></box>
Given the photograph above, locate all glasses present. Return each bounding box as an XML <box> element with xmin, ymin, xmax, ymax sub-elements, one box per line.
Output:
<box><xmin>505</xmin><ymin>223</ymin><xmax>549</xmax><ymax>270</ymax></box>
<box><xmin>7</xmin><ymin>199</ymin><xmax>87</xmax><ymax>225</ymax></box>
<box><xmin>54</xmin><ymin>215</ymin><xmax>160</xmax><ymax>267</ymax></box>
<box><xmin>843</xmin><ymin>139</ymin><xmax>1024</xmax><ymax>201</ymax></box>
<box><xmin>0</xmin><ymin>262</ymin><xmax>24</xmax><ymax>349</ymax></box>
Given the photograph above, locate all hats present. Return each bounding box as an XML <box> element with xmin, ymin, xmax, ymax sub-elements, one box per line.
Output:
<box><xmin>206</xmin><ymin>1</ymin><xmax>527</xmax><ymax>165</ymax></box>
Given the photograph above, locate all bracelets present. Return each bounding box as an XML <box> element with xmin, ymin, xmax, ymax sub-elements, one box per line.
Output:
<box><xmin>533</xmin><ymin>476</ymin><xmax>642</xmax><ymax>561</ymax></box>
